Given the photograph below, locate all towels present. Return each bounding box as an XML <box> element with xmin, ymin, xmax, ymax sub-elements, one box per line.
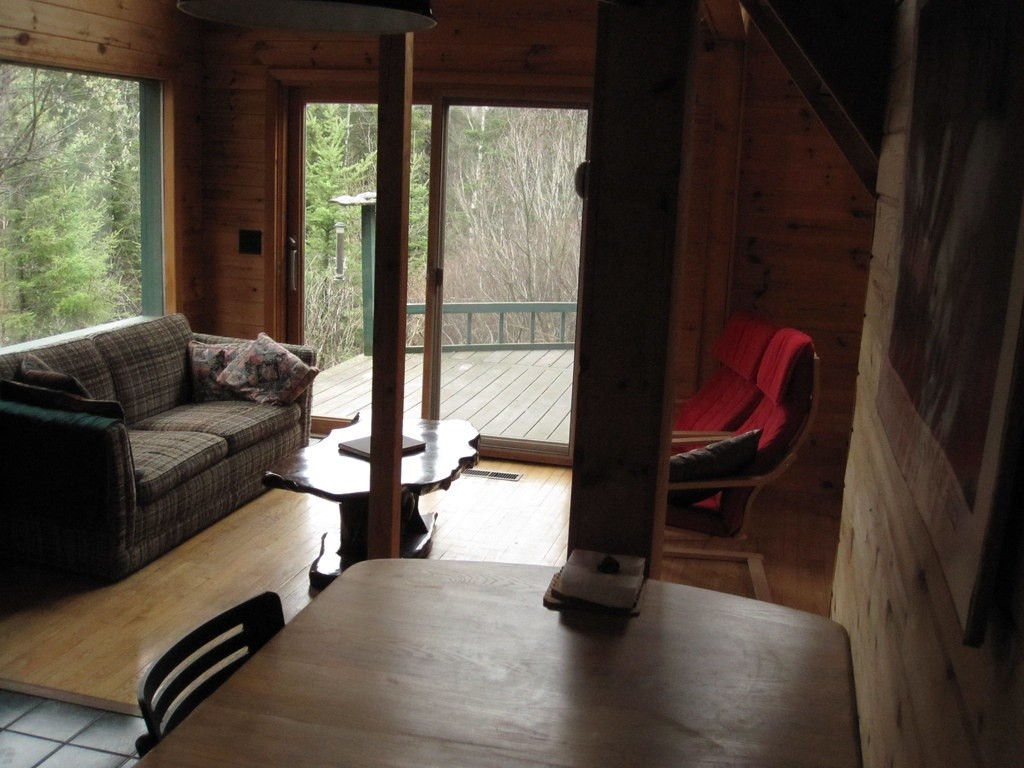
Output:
<box><xmin>559</xmin><ymin>549</ymin><xmax>645</xmax><ymax>608</ymax></box>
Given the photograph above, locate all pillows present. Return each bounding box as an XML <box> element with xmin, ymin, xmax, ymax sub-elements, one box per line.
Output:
<box><xmin>188</xmin><ymin>341</ymin><xmax>252</xmax><ymax>400</ymax></box>
<box><xmin>215</xmin><ymin>331</ymin><xmax>320</xmax><ymax>408</ymax></box>
<box><xmin>667</xmin><ymin>426</ymin><xmax>763</xmax><ymax>507</ymax></box>
<box><xmin>22</xmin><ymin>353</ymin><xmax>94</xmax><ymax>399</ymax></box>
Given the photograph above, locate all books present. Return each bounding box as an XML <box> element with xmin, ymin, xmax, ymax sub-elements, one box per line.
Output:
<box><xmin>338</xmin><ymin>434</ymin><xmax>427</xmax><ymax>460</ymax></box>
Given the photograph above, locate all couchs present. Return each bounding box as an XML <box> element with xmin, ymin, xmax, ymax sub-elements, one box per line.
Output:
<box><xmin>0</xmin><ymin>314</ymin><xmax>320</xmax><ymax>585</ymax></box>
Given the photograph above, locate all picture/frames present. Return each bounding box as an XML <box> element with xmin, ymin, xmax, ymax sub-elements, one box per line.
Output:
<box><xmin>874</xmin><ymin>1</ymin><xmax>1024</xmax><ymax>652</ymax></box>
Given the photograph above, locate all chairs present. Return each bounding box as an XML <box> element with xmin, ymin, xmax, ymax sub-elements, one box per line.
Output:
<box><xmin>136</xmin><ymin>592</ymin><xmax>286</xmax><ymax>757</ymax></box>
<box><xmin>665</xmin><ymin>308</ymin><xmax>820</xmax><ymax>540</ymax></box>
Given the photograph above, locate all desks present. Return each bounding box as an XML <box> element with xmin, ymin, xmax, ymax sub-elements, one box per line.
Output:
<box><xmin>130</xmin><ymin>558</ymin><xmax>864</xmax><ymax>768</ymax></box>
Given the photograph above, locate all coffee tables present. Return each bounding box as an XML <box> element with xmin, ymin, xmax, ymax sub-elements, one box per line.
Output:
<box><xmin>261</xmin><ymin>406</ymin><xmax>481</xmax><ymax>590</ymax></box>
